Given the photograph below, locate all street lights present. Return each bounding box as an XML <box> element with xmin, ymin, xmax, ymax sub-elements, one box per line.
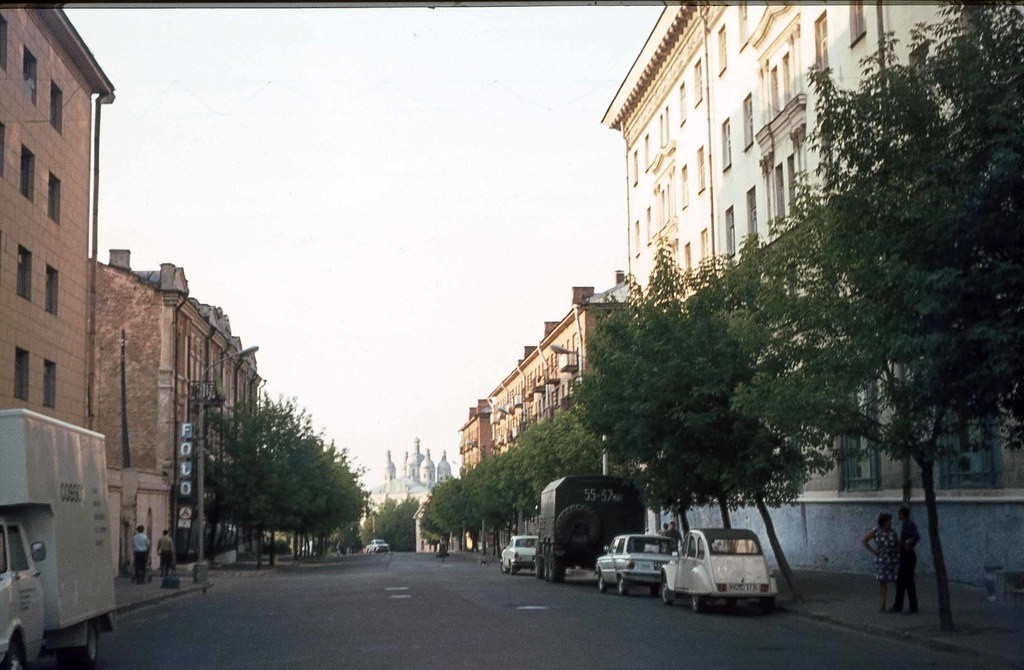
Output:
<box><xmin>550</xmin><ymin>341</ymin><xmax>612</xmax><ymax>475</ymax></box>
<box><xmin>194</xmin><ymin>345</ymin><xmax>260</xmax><ymax>582</ymax></box>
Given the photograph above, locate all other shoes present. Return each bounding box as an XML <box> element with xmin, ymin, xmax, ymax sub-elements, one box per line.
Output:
<box><xmin>879</xmin><ymin>606</ymin><xmax>918</xmax><ymax>615</ymax></box>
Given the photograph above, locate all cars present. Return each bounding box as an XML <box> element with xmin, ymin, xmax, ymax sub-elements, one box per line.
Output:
<box><xmin>365</xmin><ymin>539</ymin><xmax>390</xmax><ymax>553</ymax></box>
<box><xmin>499</xmin><ymin>534</ymin><xmax>539</xmax><ymax>577</ymax></box>
<box><xmin>596</xmin><ymin>532</ymin><xmax>676</xmax><ymax>595</ymax></box>
<box><xmin>660</xmin><ymin>527</ymin><xmax>779</xmax><ymax>614</ymax></box>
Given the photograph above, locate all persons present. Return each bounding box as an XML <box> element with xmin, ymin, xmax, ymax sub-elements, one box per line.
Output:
<box><xmin>660</xmin><ymin>522</ymin><xmax>669</xmax><ymax>536</ymax></box>
<box><xmin>435</xmin><ymin>536</ymin><xmax>449</xmax><ymax>562</ymax></box>
<box><xmin>132</xmin><ymin>526</ymin><xmax>151</xmax><ymax>584</ymax></box>
<box><xmin>156</xmin><ymin>529</ymin><xmax>174</xmax><ymax>580</ymax></box>
<box><xmin>861</xmin><ymin>512</ymin><xmax>901</xmax><ymax>613</ymax></box>
<box><xmin>664</xmin><ymin>521</ymin><xmax>683</xmax><ymax>555</ymax></box>
<box><xmin>886</xmin><ymin>506</ymin><xmax>920</xmax><ymax>613</ymax></box>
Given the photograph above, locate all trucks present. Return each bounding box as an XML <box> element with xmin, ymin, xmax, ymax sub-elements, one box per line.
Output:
<box><xmin>536</xmin><ymin>474</ymin><xmax>644</xmax><ymax>584</ymax></box>
<box><xmin>0</xmin><ymin>405</ymin><xmax>119</xmax><ymax>670</ymax></box>
